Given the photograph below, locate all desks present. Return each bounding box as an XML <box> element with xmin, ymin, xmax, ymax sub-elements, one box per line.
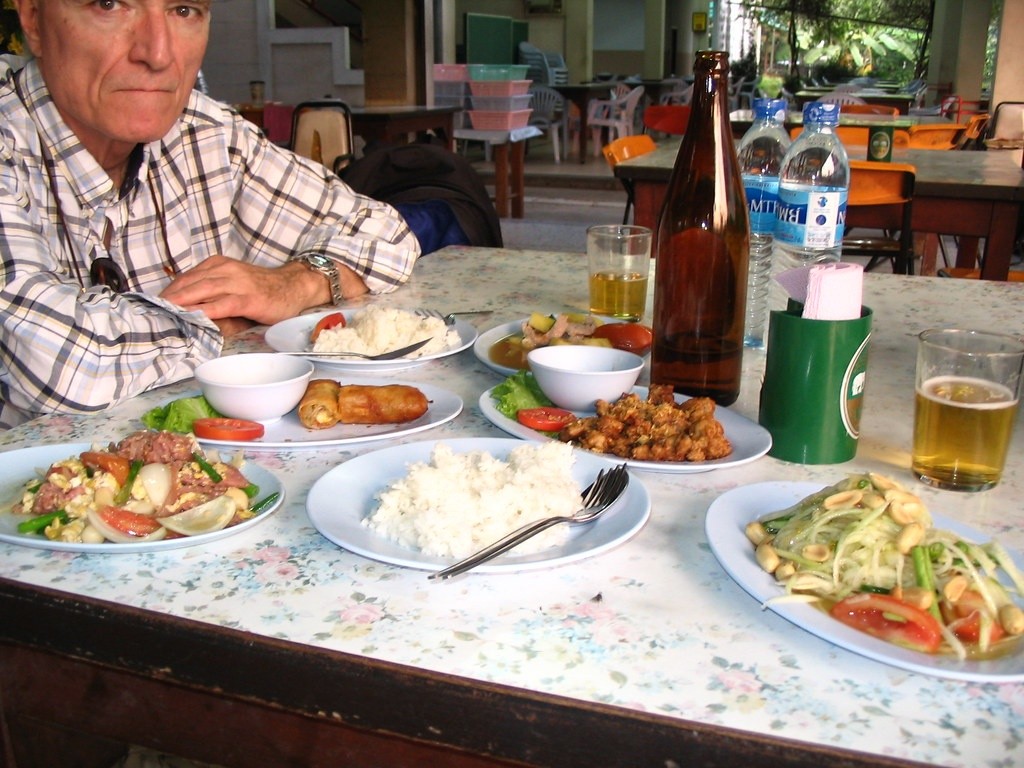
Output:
<box><xmin>453</xmin><ymin>127</ymin><xmax>543</xmax><ymax>218</ymax></box>
<box><xmin>827</xmin><ymin>83</ymin><xmax>899</xmax><ymax>88</ymax></box>
<box><xmin>352</xmin><ymin>104</ymin><xmax>464</xmax><ymax>155</ymax></box>
<box><xmin>549</xmin><ymin>83</ymin><xmax>616</xmax><ymax>165</ymax></box>
<box><xmin>803</xmin><ymin>86</ymin><xmax>894</xmax><ymax>92</ymax></box>
<box><xmin>795</xmin><ymin>91</ymin><xmax>915</xmax><ymax>115</ymax></box>
<box><xmin>611</xmin><ymin>139</ymin><xmax>1024</xmax><ymax>282</ymax></box>
<box><xmin>624</xmin><ymin>82</ymin><xmax>677</xmax><ymax>138</ymax></box>
<box><xmin>730</xmin><ymin>109</ymin><xmax>956</xmax><ymax>138</ymax></box>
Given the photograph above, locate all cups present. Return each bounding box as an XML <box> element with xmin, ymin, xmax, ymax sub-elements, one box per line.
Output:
<box><xmin>586</xmin><ymin>224</ymin><xmax>653</xmax><ymax>323</ymax></box>
<box><xmin>911</xmin><ymin>328</ymin><xmax>1024</xmax><ymax>493</ymax></box>
<box><xmin>758</xmin><ymin>305</ymin><xmax>873</xmax><ymax>465</ymax></box>
<box><xmin>250</xmin><ymin>81</ymin><xmax>266</xmax><ymax>108</ymax></box>
<box><xmin>867</xmin><ymin>127</ymin><xmax>894</xmax><ymax>163</ymax></box>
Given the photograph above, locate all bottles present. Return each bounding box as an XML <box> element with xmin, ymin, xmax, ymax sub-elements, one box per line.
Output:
<box><xmin>735</xmin><ymin>99</ymin><xmax>792</xmax><ymax>353</ymax></box>
<box><xmin>649</xmin><ymin>50</ymin><xmax>752</xmax><ymax>409</ymax></box>
<box><xmin>762</xmin><ymin>101</ymin><xmax>850</xmax><ymax>353</ymax></box>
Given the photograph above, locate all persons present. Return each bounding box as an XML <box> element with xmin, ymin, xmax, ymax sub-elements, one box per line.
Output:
<box><xmin>0</xmin><ymin>0</ymin><xmax>422</xmax><ymax>424</ymax></box>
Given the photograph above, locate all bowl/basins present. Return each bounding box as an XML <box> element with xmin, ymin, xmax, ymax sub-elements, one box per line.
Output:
<box><xmin>193</xmin><ymin>353</ymin><xmax>314</xmax><ymax>425</ymax></box>
<box><xmin>527</xmin><ymin>345</ymin><xmax>645</xmax><ymax>411</ymax></box>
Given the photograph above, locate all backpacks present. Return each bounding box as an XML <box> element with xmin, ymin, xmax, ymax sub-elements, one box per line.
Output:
<box><xmin>333</xmin><ymin>142</ymin><xmax>503</xmax><ymax>257</ymax></box>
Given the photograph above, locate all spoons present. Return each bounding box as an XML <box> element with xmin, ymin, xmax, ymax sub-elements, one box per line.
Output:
<box><xmin>443</xmin><ymin>470</ymin><xmax>629</xmax><ymax>579</ymax></box>
<box><xmin>237</xmin><ymin>337</ymin><xmax>434</xmax><ymax>360</ymax></box>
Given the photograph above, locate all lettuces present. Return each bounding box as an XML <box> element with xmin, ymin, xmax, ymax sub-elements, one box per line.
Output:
<box><xmin>489</xmin><ymin>371</ymin><xmax>560</xmax><ymax>438</ymax></box>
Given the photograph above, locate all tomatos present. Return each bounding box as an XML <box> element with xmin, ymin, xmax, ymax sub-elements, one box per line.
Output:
<box><xmin>517</xmin><ymin>408</ymin><xmax>575</xmax><ymax>431</ymax></box>
<box><xmin>311</xmin><ymin>312</ymin><xmax>347</xmax><ymax>341</ymax></box>
<box><xmin>593</xmin><ymin>323</ymin><xmax>651</xmax><ymax>349</ymax></box>
<box><xmin>830</xmin><ymin>594</ymin><xmax>940</xmax><ymax>654</ymax></box>
<box><xmin>193</xmin><ymin>418</ymin><xmax>264</xmax><ymax>441</ymax></box>
<box><xmin>98</xmin><ymin>506</ymin><xmax>162</xmax><ymax>537</ymax></box>
<box><xmin>80</xmin><ymin>451</ymin><xmax>129</xmax><ymax>485</ymax></box>
<box><xmin>956</xmin><ymin>616</ymin><xmax>1005</xmax><ymax>642</ymax></box>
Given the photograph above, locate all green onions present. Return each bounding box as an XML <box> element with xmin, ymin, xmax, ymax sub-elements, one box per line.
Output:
<box><xmin>18</xmin><ymin>453</ymin><xmax>279</xmax><ymax>534</ymax></box>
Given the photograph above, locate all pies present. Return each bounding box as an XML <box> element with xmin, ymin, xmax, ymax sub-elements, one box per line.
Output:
<box><xmin>298</xmin><ymin>379</ymin><xmax>428</xmax><ymax>430</ymax></box>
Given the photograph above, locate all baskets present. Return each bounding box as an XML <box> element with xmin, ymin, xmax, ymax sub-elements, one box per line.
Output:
<box><xmin>433</xmin><ymin>62</ymin><xmax>533</xmax><ymax>131</ymax></box>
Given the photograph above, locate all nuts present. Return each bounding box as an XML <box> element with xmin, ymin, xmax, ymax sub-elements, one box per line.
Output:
<box><xmin>745</xmin><ymin>472</ymin><xmax>1024</xmax><ymax>635</ymax></box>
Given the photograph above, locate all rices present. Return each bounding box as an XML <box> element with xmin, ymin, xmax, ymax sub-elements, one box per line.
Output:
<box><xmin>361</xmin><ymin>441</ymin><xmax>582</xmax><ymax>557</ymax></box>
<box><xmin>314</xmin><ymin>304</ymin><xmax>461</xmax><ymax>360</ymax></box>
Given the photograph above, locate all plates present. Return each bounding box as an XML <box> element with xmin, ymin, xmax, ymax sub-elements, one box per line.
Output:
<box><xmin>306</xmin><ymin>436</ymin><xmax>651</xmax><ymax>573</ymax></box>
<box><xmin>473</xmin><ymin>314</ymin><xmax>651</xmax><ymax>378</ymax></box>
<box><xmin>478</xmin><ymin>384</ymin><xmax>772</xmax><ymax>474</ymax></box>
<box><xmin>150</xmin><ymin>376</ymin><xmax>464</xmax><ymax>447</ymax></box>
<box><xmin>0</xmin><ymin>442</ymin><xmax>285</xmax><ymax>554</ymax></box>
<box><xmin>704</xmin><ymin>480</ymin><xmax>1024</xmax><ymax>684</ymax></box>
<box><xmin>265</xmin><ymin>309</ymin><xmax>479</xmax><ymax>372</ymax></box>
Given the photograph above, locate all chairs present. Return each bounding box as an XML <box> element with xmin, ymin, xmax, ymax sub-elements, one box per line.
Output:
<box><xmin>290</xmin><ymin>101</ymin><xmax>353</xmax><ymax>173</ymax></box>
<box><xmin>337</xmin><ymin>143</ymin><xmax>504</xmax><ymax>255</ymax></box>
<box><xmin>517</xmin><ymin>43</ymin><xmax>1024</xmax><ymax>281</ymax></box>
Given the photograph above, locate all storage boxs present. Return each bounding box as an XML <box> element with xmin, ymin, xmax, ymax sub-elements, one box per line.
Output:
<box><xmin>431</xmin><ymin>63</ymin><xmax>534</xmax><ymax>130</ymax></box>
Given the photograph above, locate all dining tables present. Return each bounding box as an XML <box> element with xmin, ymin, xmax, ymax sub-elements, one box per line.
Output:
<box><xmin>1</xmin><ymin>245</ymin><xmax>1024</xmax><ymax>768</ymax></box>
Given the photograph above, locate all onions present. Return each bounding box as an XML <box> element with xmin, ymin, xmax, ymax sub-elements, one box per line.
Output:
<box><xmin>82</xmin><ymin>461</ymin><xmax>249</xmax><ymax>543</ymax></box>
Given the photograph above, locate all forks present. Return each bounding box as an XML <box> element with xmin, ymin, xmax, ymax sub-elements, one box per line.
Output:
<box><xmin>415</xmin><ymin>309</ymin><xmax>493</xmax><ymax>326</ymax></box>
<box><xmin>428</xmin><ymin>463</ymin><xmax>627</xmax><ymax>580</ymax></box>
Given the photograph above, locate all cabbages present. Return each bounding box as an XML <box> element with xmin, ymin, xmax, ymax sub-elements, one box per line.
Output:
<box><xmin>141</xmin><ymin>395</ymin><xmax>221</xmax><ymax>435</ymax></box>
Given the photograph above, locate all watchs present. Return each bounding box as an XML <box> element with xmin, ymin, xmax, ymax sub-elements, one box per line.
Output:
<box><xmin>288</xmin><ymin>254</ymin><xmax>344</xmax><ymax>306</ymax></box>
